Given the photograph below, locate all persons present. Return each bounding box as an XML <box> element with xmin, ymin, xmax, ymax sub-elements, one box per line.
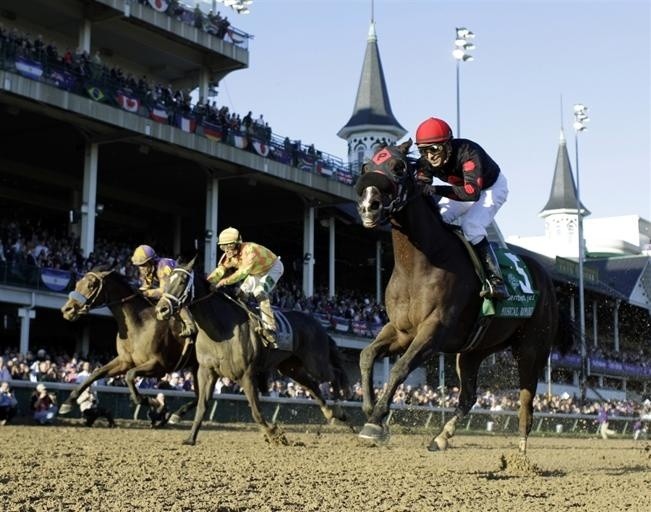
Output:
<box><xmin>140</xmin><ymin>1</ymin><xmax>184</xmax><ymax>18</ymax></box>
<box><xmin>102</xmin><ymin>64</ymin><xmax>192</xmax><ymax>116</ymax></box>
<box><xmin>415</xmin><ymin>117</ymin><xmax>510</xmax><ymax>299</ymax></box>
<box><xmin>2</xmin><ymin>23</ymin><xmax>105</xmax><ymax>82</ymax></box>
<box><xmin>552</xmin><ymin>334</ymin><xmax>651</xmax><ymax>368</ymax></box>
<box><xmin>132</xmin><ymin>244</ymin><xmax>196</xmax><ymax>338</ymax></box>
<box><xmin>192</xmin><ymin>3</ymin><xmax>230</xmax><ymax>39</ymax></box>
<box><xmin>191</xmin><ymin>96</ymin><xmax>272</xmax><ymax>142</ymax></box>
<box><xmin>205</xmin><ymin>226</ymin><xmax>284</xmax><ymax>349</ymax></box>
<box><xmin>2</xmin><ymin>346</ymin><xmax>194</xmax><ymax>427</ymax></box>
<box><xmin>284</xmin><ymin>137</ymin><xmax>315</xmax><ymax>167</ymax></box>
<box><xmin>210</xmin><ymin>367</ymin><xmax>651</xmax><ymax>436</ymax></box>
<box><xmin>253</xmin><ymin>278</ymin><xmax>389</xmax><ymax>338</ymax></box>
<box><xmin>2</xmin><ymin>216</ymin><xmax>180</xmax><ymax>287</ymax></box>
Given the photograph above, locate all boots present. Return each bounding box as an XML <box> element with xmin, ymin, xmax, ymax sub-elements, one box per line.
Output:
<box><xmin>468</xmin><ymin>236</ymin><xmax>509</xmax><ymax>300</ymax></box>
<box><xmin>176</xmin><ymin>306</ymin><xmax>197</xmax><ymax>337</ymax></box>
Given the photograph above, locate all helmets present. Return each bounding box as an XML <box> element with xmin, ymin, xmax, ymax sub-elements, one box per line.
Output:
<box><xmin>217</xmin><ymin>226</ymin><xmax>242</xmax><ymax>244</ymax></box>
<box><xmin>414</xmin><ymin>117</ymin><xmax>452</xmax><ymax>144</ymax></box>
<box><xmin>131</xmin><ymin>245</ymin><xmax>157</xmax><ymax>266</ymax></box>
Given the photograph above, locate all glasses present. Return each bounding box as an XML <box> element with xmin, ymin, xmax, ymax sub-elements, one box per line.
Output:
<box><xmin>220</xmin><ymin>244</ymin><xmax>236</xmax><ymax>250</ymax></box>
<box><xmin>419</xmin><ymin>136</ymin><xmax>453</xmax><ymax>156</ymax></box>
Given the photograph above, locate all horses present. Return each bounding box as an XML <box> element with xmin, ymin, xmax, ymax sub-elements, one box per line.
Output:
<box><xmin>155</xmin><ymin>255</ymin><xmax>351</xmax><ymax>445</ymax></box>
<box><xmin>58</xmin><ymin>260</ymin><xmax>199</xmax><ymax>427</ymax></box>
<box><xmin>354</xmin><ymin>137</ymin><xmax>584</xmax><ymax>459</ymax></box>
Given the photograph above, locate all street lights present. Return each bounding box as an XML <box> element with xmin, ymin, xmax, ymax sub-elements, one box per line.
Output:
<box><xmin>571</xmin><ymin>100</ymin><xmax>595</xmax><ymax>399</ymax></box>
<box><xmin>451</xmin><ymin>27</ymin><xmax>477</xmax><ymax>138</ymax></box>
<box><xmin>203</xmin><ymin>0</ymin><xmax>255</xmax><ymax>24</ymax></box>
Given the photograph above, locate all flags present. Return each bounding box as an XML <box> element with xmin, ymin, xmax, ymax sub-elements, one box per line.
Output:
<box><xmin>336</xmin><ymin>168</ymin><xmax>354</xmax><ymax>185</ymax></box>
<box><xmin>317</xmin><ymin>160</ymin><xmax>333</xmax><ymax>177</ymax></box>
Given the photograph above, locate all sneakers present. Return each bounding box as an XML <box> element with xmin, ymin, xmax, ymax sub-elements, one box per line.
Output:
<box><xmin>261</xmin><ymin>330</ymin><xmax>279</xmax><ymax>349</ymax></box>
